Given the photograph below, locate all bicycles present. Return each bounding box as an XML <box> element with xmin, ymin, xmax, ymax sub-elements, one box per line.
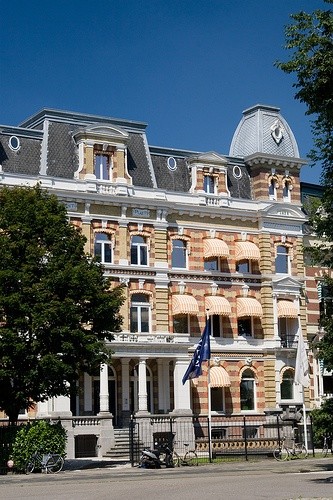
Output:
<box><xmin>24</xmin><ymin>440</ymin><xmax>64</xmax><ymax>475</ymax></box>
<box><xmin>273</xmin><ymin>436</ymin><xmax>308</xmax><ymax>461</ymax></box>
<box><xmin>167</xmin><ymin>440</ymin><xmax>198</xmax><ymax>466</ymax></box>
<box><xmin>319</xmin><ymin>433</ymin><xmax>333</xmax><ymax>458</ymax></box>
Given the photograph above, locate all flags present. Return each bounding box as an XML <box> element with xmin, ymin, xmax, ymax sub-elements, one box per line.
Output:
<box><xmin>295</xmin><ymin>324</ymin><xmax>311</xmax><ymax>388</ymax></box>
<box><xmin>182</xmin><ymin>313</ymin><xmax>212</xmax><ymax>383</ymax></box>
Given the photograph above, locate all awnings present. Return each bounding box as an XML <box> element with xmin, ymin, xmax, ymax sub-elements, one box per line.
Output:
<box><xmin>203</xmin><ymin>239</ymin><xmax>297</xmax><ymax>388</ymax></box>
<box><xmin>172</xmin><ymin>293</ymin><xmax>202</xmax><ymax>315</ymax></box>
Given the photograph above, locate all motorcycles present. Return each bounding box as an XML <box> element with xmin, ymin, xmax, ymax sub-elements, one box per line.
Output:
<box><xmin>138</xmin><ymin>437</ymin><xmax>174</xmax><ymax>468</ymax></box>
